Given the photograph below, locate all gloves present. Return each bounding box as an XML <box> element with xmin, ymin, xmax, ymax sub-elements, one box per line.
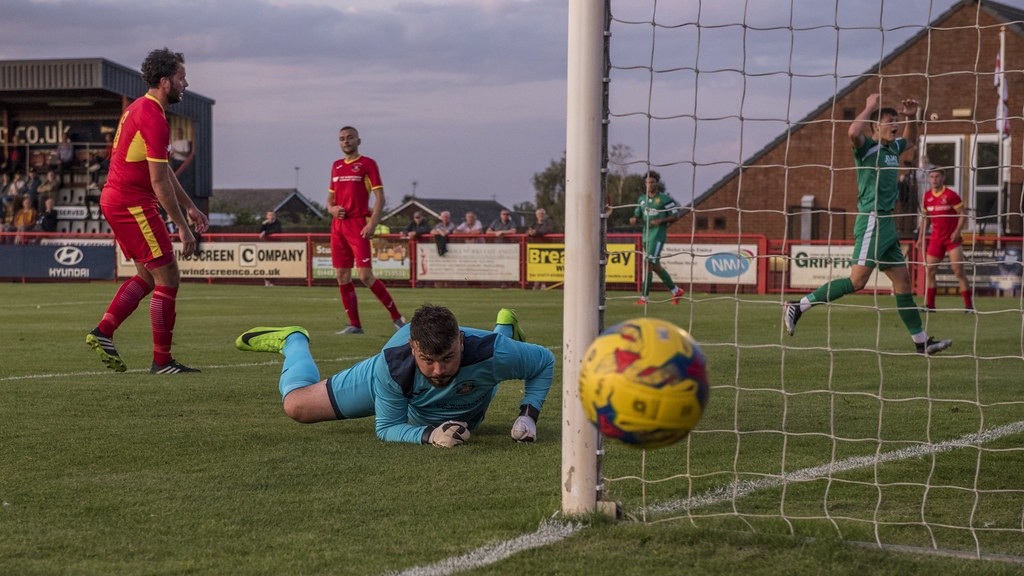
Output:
<box><xmin>510</xmin><ymin>404</ymin><xmax>540</xmax><ymax>443</ymax></box>
<box><xmin>422</xmin><ymin>421</ymin><xmax>471</xmax><ymax>449</ymax></box>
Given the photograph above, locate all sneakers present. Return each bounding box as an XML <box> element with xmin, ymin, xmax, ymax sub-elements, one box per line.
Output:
<box><xmin>784</xmin><ymin>300</ymin><xmax>802</xmax><ymax>335</ymax></box>
<box><xmin>914</xmin><ymin>336</ymin><xmax>951</xmax><ymax>355</ymax></box>
<box><xmin>150</xmin><ymin>358</ymin><xmax>201</xmax><ymax>374</ymax></box>
<box><xmin>495</xmin><ymin>308</ymin><xmax>526</xmax><ymax>342</ymax></box>
<box><xmin>86</xmin><ymin>327</ymin><xmax>127</xmax><ymax>372</ymax></box>
<box><xmin>234</xmin><ymin>326</ymin><xmax>310</xmax><ymax>354</ymax></box>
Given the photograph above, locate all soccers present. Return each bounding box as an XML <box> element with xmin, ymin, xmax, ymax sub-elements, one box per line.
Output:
<box><xmin>577</xmin><ymin>314</ymin><xmax>711</xmax><ymax>452</ymax></box>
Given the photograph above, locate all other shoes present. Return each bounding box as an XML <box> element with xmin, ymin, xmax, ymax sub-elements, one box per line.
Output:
<box><xmin>89</xmin><ymin>163</ymin><xmax>100</xmax><ymax>172</ymax></box>
<box><xmin>336</xmin><ymin>326</ymin><xmax>363</xmax><ymax>334</ymax></box>
<box><xmin>88</xmin><ymin>182</ymin><xmax>99</xmax><ymax>190</ymax></box>
<box><xmin>672</xmin><ymin>288</ymin><xmax>684</xmax><ymax>304</ymax></box>
<box><xmin>633</xmin><ymin>299</ymin><xmax>645</xmax><ymax>304</ymax></box>
<box><xmin>922</xmin><ymin>307</ymin><xmax>936</xmax><ymax>312</ymax></box>
<box><xmin>965</xmin><ymin>308</ymin><xmax>973</xmax><ymax>314</ymax></box>
<box><xmin>394</xmin><ymin>316</ymin><xmax>406</xmax><ymax>330</ymax></box>
<box><xmin>532</xmin><ymin>282</ymin><xmax>539</xmax><ymax>290</ymax></box>
<box><xmin>540</xmin><ymin>283</ymin><xmax>546</xmax><ymax>291</ymax></box>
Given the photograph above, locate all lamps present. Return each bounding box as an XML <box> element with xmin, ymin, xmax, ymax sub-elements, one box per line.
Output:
<box><xmin>952</xmin><ymin>108</ymin><xmax>972</xmax><ymax>117</ymax></box>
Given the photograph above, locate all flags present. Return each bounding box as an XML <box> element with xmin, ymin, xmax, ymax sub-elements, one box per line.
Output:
<box><xmin>994</xmin><ymin>54</ymin><xmax>1010</xmax><ymax>140</ymax></box>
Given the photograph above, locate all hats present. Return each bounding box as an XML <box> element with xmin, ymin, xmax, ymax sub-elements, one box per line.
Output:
<box><xmin>29</xmin><ymin>168</ymin><xmax>37</xmax><ymax>172</ymax></box>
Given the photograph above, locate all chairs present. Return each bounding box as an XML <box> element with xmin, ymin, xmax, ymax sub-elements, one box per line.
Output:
<box><xmin>35</xmin><ymin>149</ymin><xmax>113</xmax><ymax>234</ymax></box>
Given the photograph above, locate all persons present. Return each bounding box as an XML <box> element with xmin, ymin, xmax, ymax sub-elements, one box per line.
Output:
<box><xmin>898</xmin><ymin>160</ymin><xmax>918</xmax><ymax>235</ymax></box>
<box><xmin>403</xmin><ymin>212</ymin><xmax>433</xmax><ymax>287</ymax></box>
<box><xmin>528</xmin><ymin>208</ymin><xmax>556</xmax><ymax>290</ymax></box>
<box><xmin>452</xmin><ymin>212</ymin><xmax>486</xmax><ymax>288</ymax></box>
<box><xmin>235</xmin><ymin>303</ymin><xmax>556</xmax><ymax>450</ymax></box>
<box><xmin>86</xmin><ymin>47</ymin><xmax>210</xmax><ymax>374</ymax></box>
<box><xmin>486</xmin><ymin>208</ymin><xmax>518</xmax><ymax>288</ymax></box>
<box><xmin>630</xmin><ymin>171</ymin><xmax>684</xmax><ymax>305</ymax></box>
<box><xmin>87</xmin><ymin>134</ymin><xmax>115</xmax><ymax>190</ymax></box>
<box><xmin>165</xmin><ymin>207</ymin><xmax>201</xmax><ymax>243</ymax></box>
<box><xmin>430</xmin><ymin>211</ymin><xmax>454</xmax><ymax>288</ymax></box>
<box><xmin>258</xmin><ymin>210</ymin><xmax>281</xmax><ymax>287</ymax></box>
<box><xmin>1</xmin><ymin>133</ymin><xmax>76</xmax><ymax>189</ymax></box>
<box><xmin>327</xmin><ymin>126</ymin><xmax>407</xmax><ymax>335</ymax></box>
<box><xmin>169</xmin><ymin>128</ymin><xmax>190</xmax><ymax>172</ymax></box>
<box><xmin>916</xmin><ymin>167</ymin><xmax>976</xmax><ymax>314</ymax></box>
<box><xmin>783</xmin><ymin>94</ymin><xmax>952</xmax><ymax>356</ymax></box>
<box><xmin>0</xmin><ymin>196</ymin><xmax>58</xmax><ymax>245</ymax></box>
<box><xmin>0</xmin><ymin>167</ymin><xmax>56</xmax><ymax>204</ymax></box>
